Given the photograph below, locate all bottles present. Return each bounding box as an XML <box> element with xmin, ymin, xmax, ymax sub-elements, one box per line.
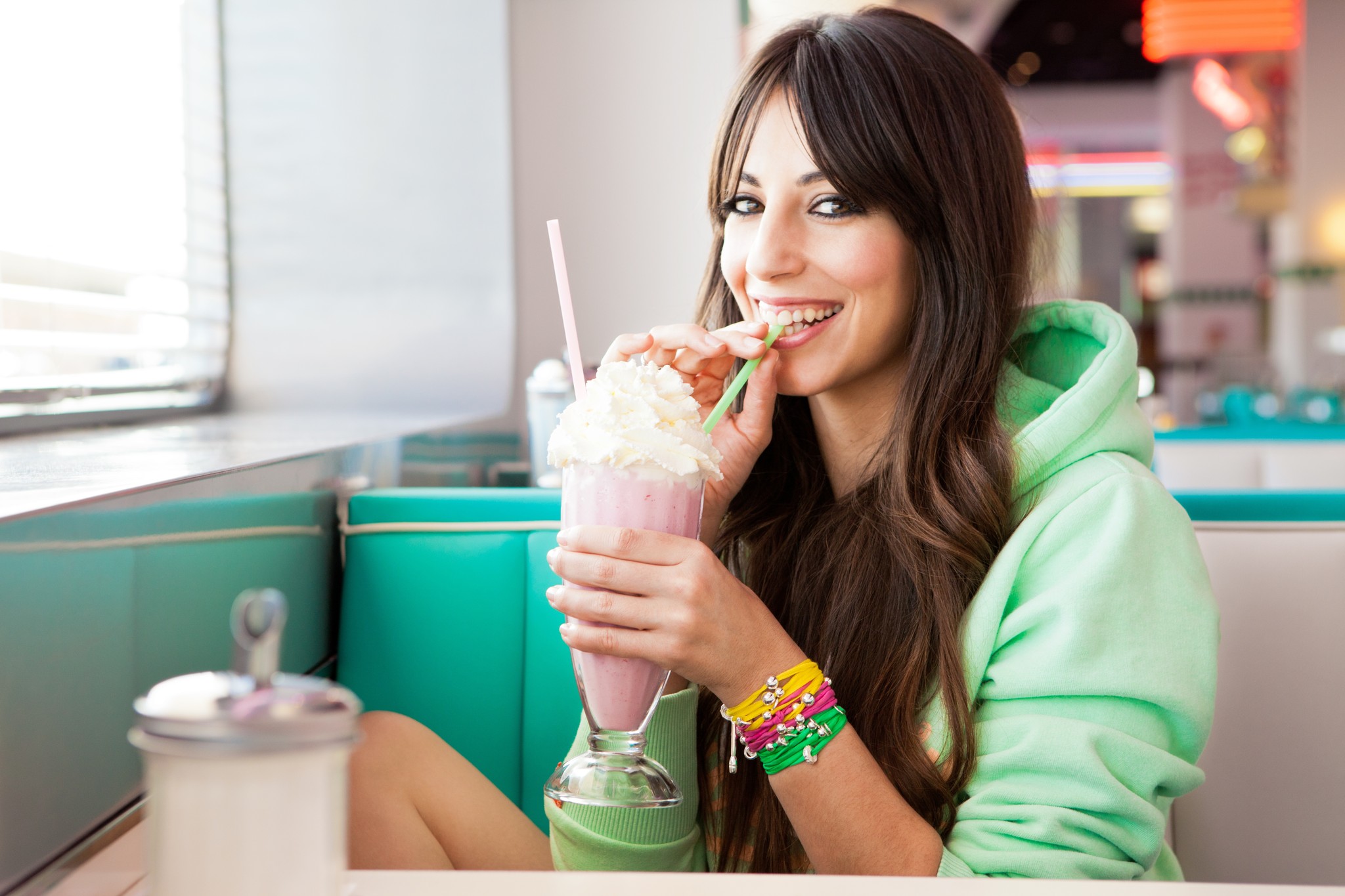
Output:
<box><xmin>116</xmin><ymin>586</ymin><xmax>364</xmax><ymax>896</ymax></box>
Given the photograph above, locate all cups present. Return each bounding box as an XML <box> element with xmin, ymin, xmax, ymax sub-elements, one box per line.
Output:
<box><xmin>538</xmin><ymin>465</ymin><xmax>710</xmax><ymax>813</ymax></box>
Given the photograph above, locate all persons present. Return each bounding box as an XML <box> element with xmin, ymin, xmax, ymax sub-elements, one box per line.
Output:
<box><xmin>340</xmin><ymin>10</ymin><xmax>1222</xmax><ymax>880</ymax></box>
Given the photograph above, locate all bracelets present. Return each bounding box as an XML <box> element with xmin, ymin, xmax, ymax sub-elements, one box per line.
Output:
<box><xmin>723</xmin><ymin>656</ymin><xmax>848</xmax><ymax>780</ymax></box>
